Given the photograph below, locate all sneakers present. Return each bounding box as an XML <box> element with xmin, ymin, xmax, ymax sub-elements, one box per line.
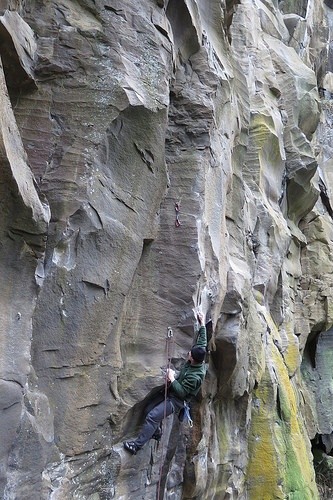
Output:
<box><xmin>150</xmin><ymin>433</ymin><xmax>162</xmax><ymax>440</ymax></box>
<box><xmin>123</xmin><ymin>441</ymin><xmax>139</xmax><ymax>455</ymax></box>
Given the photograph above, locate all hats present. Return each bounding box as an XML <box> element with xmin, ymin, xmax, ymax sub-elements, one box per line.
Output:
<box><xmin>191</xmin><ymin>347</ymin><xmax>205</xmax><ymax>362</ymax></box>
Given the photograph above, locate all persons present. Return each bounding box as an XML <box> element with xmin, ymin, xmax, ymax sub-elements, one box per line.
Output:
<box><xmin>123</xmin><ymin>311</ymin><xmax>208</xmax><ymax>456</ymax></box>
